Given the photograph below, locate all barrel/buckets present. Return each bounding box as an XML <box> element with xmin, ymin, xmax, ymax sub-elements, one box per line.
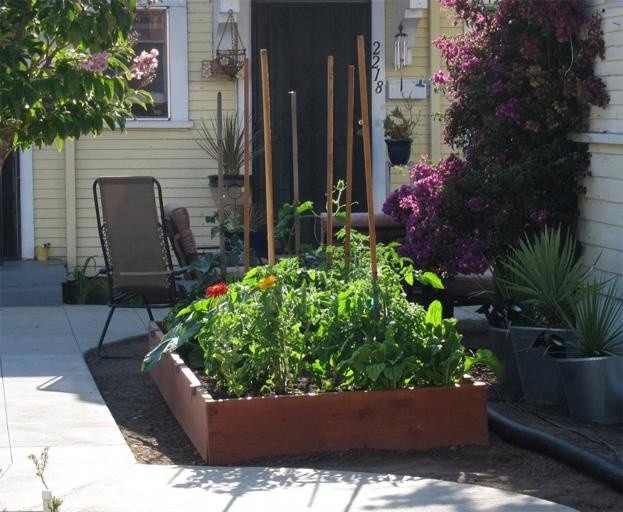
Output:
<box><xmin>508</xmin><ymin>325</ymin><xmax>572</xmax><ymax>402</ymax></box>
<box><xmin>489</xmin><ymin>327</ymin><xmax>521</xmax><ymax>394</ymax></box>
<box><xmin>558</xmin><ymin>355</ymin><xmax>623</xmax><ymax>425</ymax></box>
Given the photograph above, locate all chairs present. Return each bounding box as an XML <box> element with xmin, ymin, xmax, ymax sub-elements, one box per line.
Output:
<box><xmin>320</xmin><ymin>211</ymin><xmax>495</xmax><ymax>320</ymax></box>
<box><xmin>93</xmin><ymin>176</ymin><xmax>263</xmax><ymax>359</ymax></box>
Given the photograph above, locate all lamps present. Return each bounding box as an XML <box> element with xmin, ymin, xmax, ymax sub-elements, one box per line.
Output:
<box><xmin>215</xmin><ymin>9</ymin><xmax>247</xmax><ymax>80</ymax></box>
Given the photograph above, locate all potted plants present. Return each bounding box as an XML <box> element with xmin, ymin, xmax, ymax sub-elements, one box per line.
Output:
<box><xmin>383</xmin><ymin>90</ymin><xmax>421</xmax><ymax>166</ymax></box>
<box><xmin>36</xmin><ymin>243</ymin><xmax>51</xmax><ymax>261</ymax></box>
<box><xmin>466</xmin><ymin>222</ymin><xmax>623</xmax><ymax>424</ymax></box>
<box><xmin>192</xmin><ymin>110</ymin><xmax>265</xmax><ymax>222</ymax></box>
